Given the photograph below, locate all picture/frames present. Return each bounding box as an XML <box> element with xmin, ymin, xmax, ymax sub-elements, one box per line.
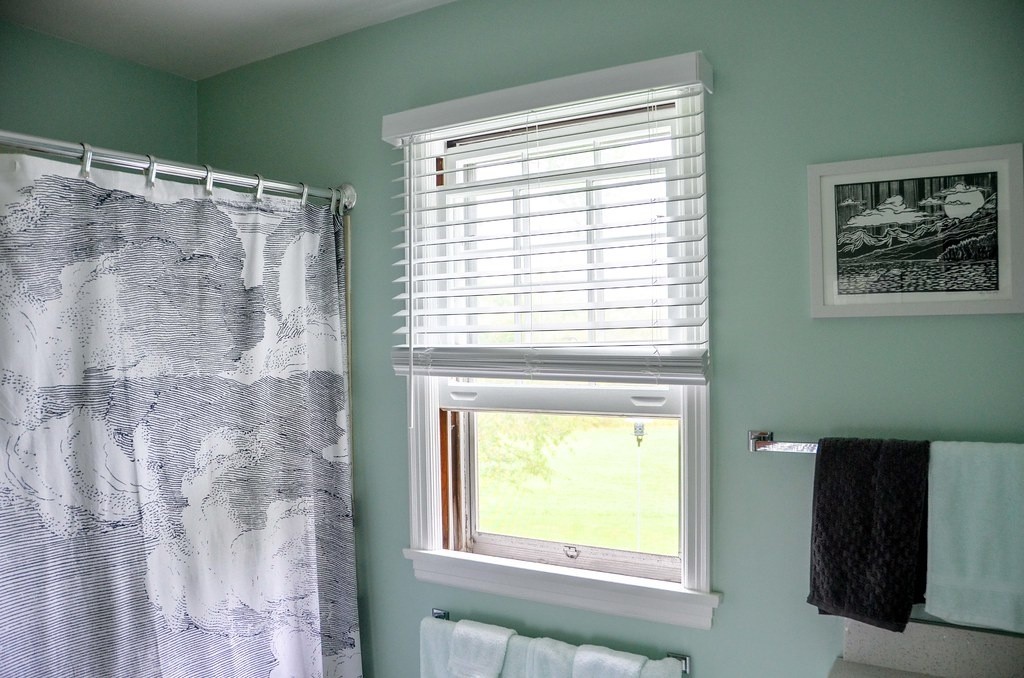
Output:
<box><xmin>805</xmin><ymin>143</ymin><xmax>1024</xmax><ymax>318</ymax></box>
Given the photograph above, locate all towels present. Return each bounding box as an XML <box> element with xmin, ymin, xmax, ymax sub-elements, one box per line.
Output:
<box><xmin>419</xmin><ymin>616</ymin><xmax>682</xmax><ymax>678</ymax></box>
<box><xmin>924</xmin><ymin>441</ymin><xmax>1024</xmax><ymax>634</ymax></box>
<box><xmin>806</xmin><ymin>438</ymin><xmax>930</xmax><ymax>633</ymax></box>
